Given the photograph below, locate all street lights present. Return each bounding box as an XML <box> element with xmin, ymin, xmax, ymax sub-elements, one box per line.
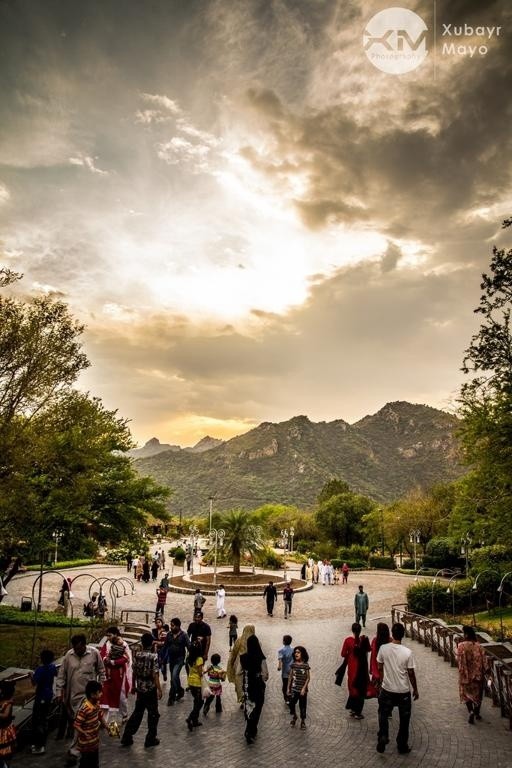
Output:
<box><xmin>460</xmin><ymin>529</ymin><xmax>471</xmax><ymax>579</ymax></box>
<box><xmin>28</xmin><ymin>569</ymin><xmax>73</xmax><ymax>666</ymax></box>
<box><xmin>186</xmin><ymin>519</ymin><xmax>295</xmax><ymax>584</ymax></box>
<box><xmin>446</xmin><ymin>574</ymin><xmax>476</xmax><ymax>626</ymax></box>
<box><xmin>409</xmin><ymin>527</ymin><xmax>421</xmax><ymax>570</ymax></box>
<box><xmin>413</xmin><ymin>566</ymin><xmax>436</xmax><ymax>615</ymax></box>
<box><xmin>431</xmin><ymin>569</ymin><xmax>456</xmax><ymax>620</ymax></box>
<box><xmin>471</xmin><ymin>570</ymin><xmax>504</xmax><ymax>641</ymax></box>
<box><xmin>66</xmin><ymin>574</ymin><xmax>136</xmax><ymax>648</ymax></box>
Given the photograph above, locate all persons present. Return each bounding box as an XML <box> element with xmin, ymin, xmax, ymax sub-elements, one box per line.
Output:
<box><xmin>227</xmin><ymin>615</ymin><xmax>269</xmax><ymax>746</ymax></box>
<box><xmin>298</xmin><ymin>556</ymin><xmax>351</xmax><ymax>587</ymax></box>
<box><xmin>215</xmin><ymin>585</ymin><xmax>227</xmax><ymax>618</ymax></box>
<box><xmin>127</xmin><ymin>547</ymin><xmax>166</xmax><ymax>582</ymax></box>
<box><xmin>151</xmin><ymin>611</ymin><xmax>226</xmax><ymax>731</ymax></box>
<box><xmin>0</xmin><ymin>682</ymin><xmax>16</xmax><ymax>767</ymax></box>
<box><xmin>262</xmin><ymin>581</ymin><xmax>277</xmax><ymax>618</ymax></box>
<box><xmin>160</xmin><ymin>574</ymin><xmax>169</xmax><ymax>591</ymax></box>
<box><xmin>29</xmin><ymin>625</ymin><xmax>133</xmax><ymax>768</ymax></box>
<box><xmin>341</xmin><ymin>620</ymin><xmax>419</xmax><ymax>756</ymax></box>
<box><xmin>193</xmin><ymin>588</ymin><xmax>206</xmax><ymax>621</ymax></box>
<box><xmin>85</xmin><ymin>592</ymin><xmax>107</xmax><ymax>620</ymax></box>
<box><xmin>152</xmin><ymin>584</ymin><xmax>167</xmax><ymax>621</ymax></box>
<box><xmin>277</xmin><ymin>635</ymin><xmax>310</xmax><ymax>731</ymax></box>
<box><xmin>282</xmin><ymin>583</ymin><xmax>294</xmax><ymax>619</ymax></box>
<box><xmin>354</xmin><ymin>585</ymin><xmax>369</xmax><ymax>627</ymax></box>
<box><xmin>58</xmin><ymin>578</ymin><xmax>72</xmax><ymax>605</ymax></box>
<box><xmin>121</xmin><ymin>633</ymin><xmax>162</xmax><ymax>747</ymax></box>
<box><xmin>179</xmin><ymin>539</ymin><xmax>198</xmax><ymax>572</ymax></box>
<box><xmin>457</xmin><ymin>625</ymin><xmax>489</xmax><ymax>725</ymax></box>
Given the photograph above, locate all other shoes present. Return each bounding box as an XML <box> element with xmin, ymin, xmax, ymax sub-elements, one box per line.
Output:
<box><xmin>144</xmin><ymin>738</ymin><xmax>159</xmax><ymax>748</ymax></box>
<box><xmin>376</xmin><ymin>737</ymin><xmax>387</xmax><ymax>752</ymax></box>
<box><xmin>349</xmin><ymin>713</ymin><xmax>364</xmax><ymax>719</ymax></box>
<box><xmin>193</xmin><ymin>722</ymin><xmax>202</xmax><ymax>727</ymax></box>
<box><xmin>123</xmin><ymin>740</ymin><xmax>134</xmax><ymax>746</ymax></box>
<box><xmin>243</xmin><ymin>732</ymin><xmax>256</xmax><ymax>744</ymax></box>
<box><xmin>290</xmin><ymin>716</ymin><xmax>297</xmax><ymax>726</ymax></box>
<box><xmin>400</xmin><ymin>749</ymin><xmax>411</xmax><ymax>752</ymax></box>
<box><xmin>301</xmin><ymin>721</ymin><xmax>307</xmax><ymax>730</ymax></box>
<box><xmin>185</xmin><ymin>718</ymin><xmax>193</xmax><ymax>731</ymax></box>
